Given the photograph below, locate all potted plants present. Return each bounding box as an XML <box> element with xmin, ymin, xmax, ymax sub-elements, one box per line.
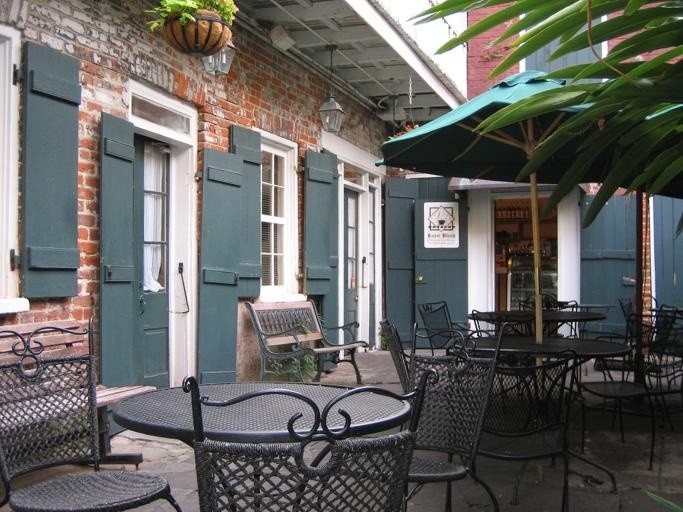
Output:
<box><xmin>140</xmin><ymin>0</ymin><xmax>241</xmax><ymax>59</ymax></box>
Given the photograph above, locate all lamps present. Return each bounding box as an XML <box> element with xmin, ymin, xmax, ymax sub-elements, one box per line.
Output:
<box><xmin>317</xmin><ymin>43</ymin><xmax>348</xmax><ymax>133</ymax></box>
<box><xmin>201</xmin><ymin>41</ymin><xmax>239</xmax><ymax>78</ymax></box>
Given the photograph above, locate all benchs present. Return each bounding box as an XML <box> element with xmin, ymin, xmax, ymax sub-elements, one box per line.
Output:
<box><xmin>244</xmin><ymin>296</ymin><xmax>369</xmax><ymax>383</ymax></box>
<box><xmin>1</xmin><ymin>308</ymin><xmax>157</xmax><ymax>501</ymax></box>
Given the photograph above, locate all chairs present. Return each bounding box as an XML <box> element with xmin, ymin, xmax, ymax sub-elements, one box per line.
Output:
<box><xmin>183</xmin><ymin>369</ymin><xmax>439</xmax><ymax>509</ymax></box>
<box><xmin>318</xmin><ymin>319</ymin><xmax>515</xmax><ymax>511</ymax></box>
<box><xmin>2</xmin><ymin>316</ymin><xmax>183</xmax><ymax>512</ymax></box>
<box><xmin>380</xmin><ymin>293</ymin><xmax>683</xmax><ymax>512</ymax></box>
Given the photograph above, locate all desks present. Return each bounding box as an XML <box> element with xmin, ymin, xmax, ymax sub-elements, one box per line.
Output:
<box><xmin>114</xmin><ymin>382</ymin><xmax>412</xmax><ymax>511</ymax></box>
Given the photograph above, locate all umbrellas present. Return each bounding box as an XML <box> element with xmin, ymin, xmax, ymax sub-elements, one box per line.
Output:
<box><xmin>371</xmin><ymin>70</ymin><xmax>683</xmax><ymax>415</ymax></box>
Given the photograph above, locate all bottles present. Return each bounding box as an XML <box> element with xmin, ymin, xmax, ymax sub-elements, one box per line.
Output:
<box><xmin>495</xmin><ymin>206</ymin><xmax>530</xmax><ymax>219</ymax></box>
